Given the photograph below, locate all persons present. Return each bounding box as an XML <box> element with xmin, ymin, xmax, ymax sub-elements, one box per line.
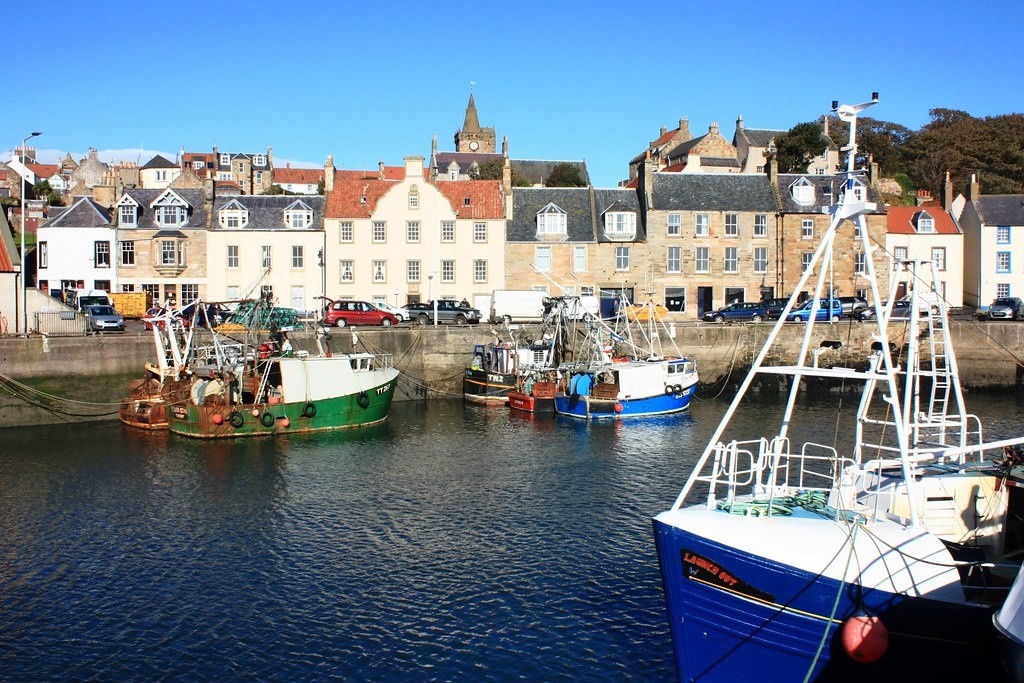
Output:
<box><xmin>460</xmin><ymin>298</ymin><xmax>470</xmax><ymax>308</ymax></box>
<box><xmin>1001</xmin><ymin>446</ymin><xmax>1020</xmax><ymax>470</ymax></box>
<box><xmin>614</xmin><ymin>300</ymin><xmax>625</xmax><ymax>326</ymax></box>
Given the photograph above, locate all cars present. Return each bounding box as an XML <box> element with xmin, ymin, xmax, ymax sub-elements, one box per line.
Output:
<box><xmin>989</xmin><ymin>297</ymin><xmax>1024</xmax><ymax>320</ymax></box>
<box><xmin>401</xmin><ymin>303</ymin><xmax>429</xmax><ymax>316</ymax></box>
<box><xmin>901</xmin><ymin>293</ymin><xmax>949</xmax><ymax>314</ymax></box>
<box><xmin>854</xmin><ymin>300</ymin><xmax>914</xmax><ymax>322</ymax></box>
<box><xmin>371</xmin><ymin>300</ymin><xmax>411</xmax><ymax>323</ymax></box>
<box><xmin>80</xmin><ymin>304</ymin><xmax>126</xmax><ymax>333</ymax></box>
<box><xmin>312</xmin><ymin>295</ymin><xmax>399</xmax><ymax>327</ymax></box>
<box><xmin>144</xmin><ymin>306</ymin><xmax>227</xmax><ymax>330</ymax></box>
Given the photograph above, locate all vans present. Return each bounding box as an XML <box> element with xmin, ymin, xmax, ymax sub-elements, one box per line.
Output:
<box><xmin>564</xmin><ymin>294</ymin><xmax>599</xmax><ymax>322</ymax></box>
<box><xmin>73</xmin><ymin>289</ymin><xmax>113</xmax><ymax>317</ymax></box>
<box><xmin>487</xmin><ymin>289</ymin><xmax>556</xmax><ymax>324</ymax></box>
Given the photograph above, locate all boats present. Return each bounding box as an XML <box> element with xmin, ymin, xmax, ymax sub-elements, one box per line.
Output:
<box><xmin>117</xmin><ymin>260</ymin><xmax>402</xmax><ymax>438</ymax></box>
<box><xmin>460</xmin><ymin>291</ymin><xmax>701</xmax><ymax>419</ymax></box>
<box><xmin>653</xmin><ymin>90</ymin><xmax>1024</xmax><ymax>683</ymax></box>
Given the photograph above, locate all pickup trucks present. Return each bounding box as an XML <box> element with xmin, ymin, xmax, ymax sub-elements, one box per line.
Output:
<box><xmin>408</xmin><ymin>299</ymin><xmax>483</xmax><ymax>325</ymax></box>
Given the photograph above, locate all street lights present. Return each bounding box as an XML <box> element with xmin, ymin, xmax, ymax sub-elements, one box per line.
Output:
<box><xmin>20</xmin><ymin>131</ymin><xmax>45</xmax><ymax>332</ymax></box>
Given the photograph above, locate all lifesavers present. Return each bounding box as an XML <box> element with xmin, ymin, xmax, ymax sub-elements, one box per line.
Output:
<box><xmin>260</xmin><ymin>412</ymin><xmax>274</xmax><ymax>427</ymax></box>
<box><xmin>674</xmin><ymin>383</ymin><xmax>683</xmax><ymax>395</ymax></box>
<box><xmin>358</xmin><ymin>393</ymin><xmax>370</xmax><ymax>407</ymax></box>
<box><xmin>229</xmin><ymin>412</ymin><xmax>245</xmax><ymax>428</ymax></box>
<box><xmin>303</xmin><ymin>403</ymin><xmax>316</xmax><ymax>418</ymax></box>
<box><xmin>664</xmin><ymin>385</ymin><xmax>674</xmax><ymax>396</ymax></box>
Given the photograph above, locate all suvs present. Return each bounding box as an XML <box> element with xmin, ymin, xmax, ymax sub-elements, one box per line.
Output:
<box><xmin>787</xmin><ymin>298</ymin><xmax>845</xmax><ymax>324</ymax></box>
<box><xmin>833</xmin><ymin>296</ymin><xmax>868</xmax><ymax>317</ymax></box>
<box><xmin>702</xmin><ymin>301</ymin><xmax>770</xmax><ymax>323</ymax></box>
<box><xmin>757</xmin><ymin>297</ymin><xmax>790</xmax><ymax>319</ymax></box>
